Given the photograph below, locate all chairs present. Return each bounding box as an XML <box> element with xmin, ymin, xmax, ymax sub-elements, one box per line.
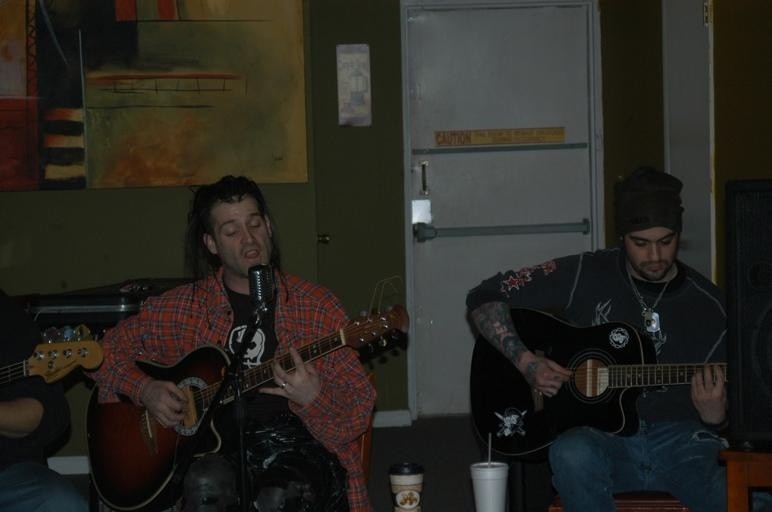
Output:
<box><xmin>548</xmin><ymin>485</ymin><xmax>694</xmax><ymax>512</ymax></box>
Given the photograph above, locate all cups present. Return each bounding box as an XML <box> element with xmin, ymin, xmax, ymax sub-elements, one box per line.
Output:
<box><xmin>388</xmin><ymin>462</ymin><xmax>424</xmax><ymax>512</ymax></box>
<box><xmin>470</xmin><ymin>462</ymin><xmax>509</xmax><ymax>512</ymax></box>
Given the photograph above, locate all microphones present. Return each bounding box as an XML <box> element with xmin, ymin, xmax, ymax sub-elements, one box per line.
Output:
<box><xmin>243</xmin><ymin>259</ymin><xmax>274</xmax><ymax>310</ymax></box>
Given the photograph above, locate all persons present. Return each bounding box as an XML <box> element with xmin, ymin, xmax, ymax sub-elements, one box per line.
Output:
<box><xmin>97</xmin><ymin>176</ymin><xmax>380</xmax><ymax>509</ymax></box>
<box><xmin>0</xmin><ymin>285</ymin><xmax>91</xmax><ymax>511</ymax></box>
<box><xmin>465</xmin><ymin>167</ymin><xmax>770</xmax><ymax>510</ymax></box>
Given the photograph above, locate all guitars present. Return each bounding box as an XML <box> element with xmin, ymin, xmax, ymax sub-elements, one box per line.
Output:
<box><xmin>86</xmin><ymin>303</ymin><xmax>409</xmax><ymax>511</ymax></box>
<box><xmin>470</xmin><ymin>306</ymin><xmax>727</xmax><ymax>456</ymax></box>
<box><xmin>0</xmin><ymin>324</ymin><xmax>103</xmax><ymax>385</ymax></box>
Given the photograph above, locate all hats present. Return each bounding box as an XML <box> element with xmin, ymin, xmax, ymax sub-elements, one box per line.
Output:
<box><xmin>613</xmin><ymin>165</ymin><xmax>683</xmax><ymax>238</ymax></box>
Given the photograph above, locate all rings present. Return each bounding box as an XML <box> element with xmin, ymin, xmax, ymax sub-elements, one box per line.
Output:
<box><xmin>280</xmin><ymin>380</ymin><xmax>288</xmax><ymax>390</ymax></box>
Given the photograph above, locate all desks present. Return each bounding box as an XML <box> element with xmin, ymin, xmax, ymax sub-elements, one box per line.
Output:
<box><xmin>719</xmin><ymin>448</ymin><xmax>772</xmax><ymax>512</ymax></box>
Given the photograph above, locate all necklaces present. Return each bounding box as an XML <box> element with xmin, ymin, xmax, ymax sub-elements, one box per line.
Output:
<box><xmin>623</xmin><ymin>253</ymin><xmax>675</xmax><ymax>326</ymax></box>
